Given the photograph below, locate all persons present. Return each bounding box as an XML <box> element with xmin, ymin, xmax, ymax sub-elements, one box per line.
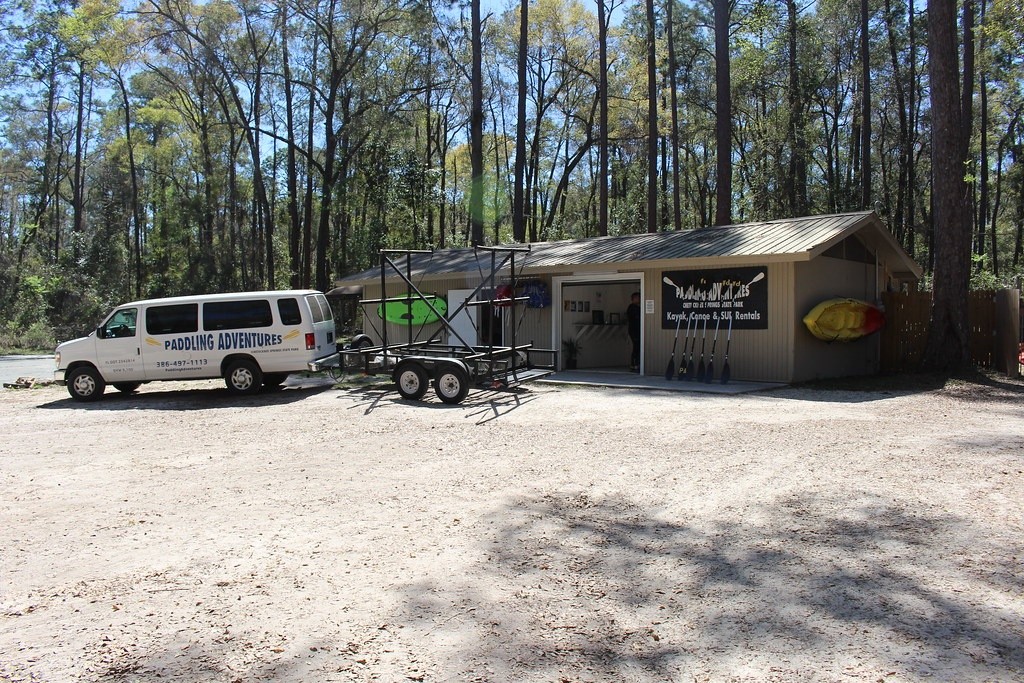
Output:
<box><xmin>628</xmin><ymin>292</ymin><xmax>640</xmax><ymax>373</ymax></box>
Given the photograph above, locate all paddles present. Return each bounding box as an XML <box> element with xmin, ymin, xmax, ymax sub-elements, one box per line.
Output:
<box><xmin>665</xmin><ymin>277</ymin><xmax>741</xmax><ymax>384</ymax></box>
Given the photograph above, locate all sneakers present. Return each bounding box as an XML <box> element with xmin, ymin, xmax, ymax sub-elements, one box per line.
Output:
<box><xmin>630</xmin><ymin>364</ymin><xmax>639</xmax><ymax>369</ymax></box>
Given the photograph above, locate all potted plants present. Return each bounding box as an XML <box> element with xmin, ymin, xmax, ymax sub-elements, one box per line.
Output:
<box><xmin>562</xmin><ymin>338</ymin><xmax>583</xmax><ymax>369</ymax></box>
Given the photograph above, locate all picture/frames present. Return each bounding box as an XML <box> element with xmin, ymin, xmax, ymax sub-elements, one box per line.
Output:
<box><xmin>610</xmin><ymin>312</ymin><xmax>620</xmax><ymax>324</ymax></box>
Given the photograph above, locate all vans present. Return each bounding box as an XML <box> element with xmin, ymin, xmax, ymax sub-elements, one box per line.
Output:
<box><xmin>53</xmin><ymin>289</ymin><xmax>340</xmax><ymax>402</ymax></box>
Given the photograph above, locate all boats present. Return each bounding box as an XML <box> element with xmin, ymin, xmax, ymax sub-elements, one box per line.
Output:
<box><xmin>377</xmin><ymin>292</ymin><xmax>447</xmax><ymax>326</ymax></box>
<box><xmin>803</xmin><ymin>298</ymin><xmax>886</xmax><ymax>342</ymax></box>
